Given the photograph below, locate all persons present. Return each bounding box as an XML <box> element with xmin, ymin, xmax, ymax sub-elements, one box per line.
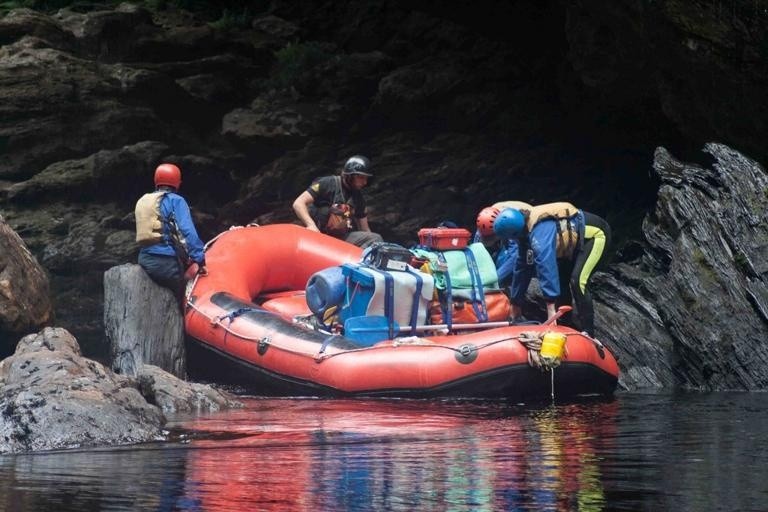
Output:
<box><xmin>494</xmin><ymin>201</ymin><xmax>613</xmax><ymax>337</ymax></box>
<box><xmin>471</xmin><ymin>199</ymin><xmax>534</xmax><ymax>288</ymax></box>
<box><xmin>291</xmin><ymin>155</ymin><xmax>384</xmax><ymax>250</ymax></box>
<box><xmin>134</xmin><ymin>162</ymin><xmax>211</xmax><ymax>383</ymax></box>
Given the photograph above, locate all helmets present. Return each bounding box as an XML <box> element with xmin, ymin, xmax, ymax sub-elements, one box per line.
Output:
<box><xmin>154</xmin><ymin>164</ymin><xmax>181</xmax><ymax>191</ymax></box>
<box><xmin>476</xmin><ymin>207</ymin><xmax>525</xmax><ymax>239</ymax></box>
<box><xmin>343</xmin><ymin>155</ymin><xmax>373</xmax><ymax>177</ymax></box>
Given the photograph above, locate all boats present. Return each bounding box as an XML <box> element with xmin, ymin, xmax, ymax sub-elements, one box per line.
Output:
<box><xmin>181</xmin><ymin>222</ymin><xmax>619</xmax><ymax>403</ymax></box>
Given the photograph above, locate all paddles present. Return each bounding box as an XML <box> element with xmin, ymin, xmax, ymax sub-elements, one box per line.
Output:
<box><xmin>344</xmin><ymin>315</ymin><xmax>542</xmax><ymax>346</ymax></box>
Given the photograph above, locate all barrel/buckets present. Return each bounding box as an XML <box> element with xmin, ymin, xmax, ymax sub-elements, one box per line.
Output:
<box><xmin>306</xmin><ymin>266</ymin><xmax>346</xmax><ymax>318</ymax></box>
